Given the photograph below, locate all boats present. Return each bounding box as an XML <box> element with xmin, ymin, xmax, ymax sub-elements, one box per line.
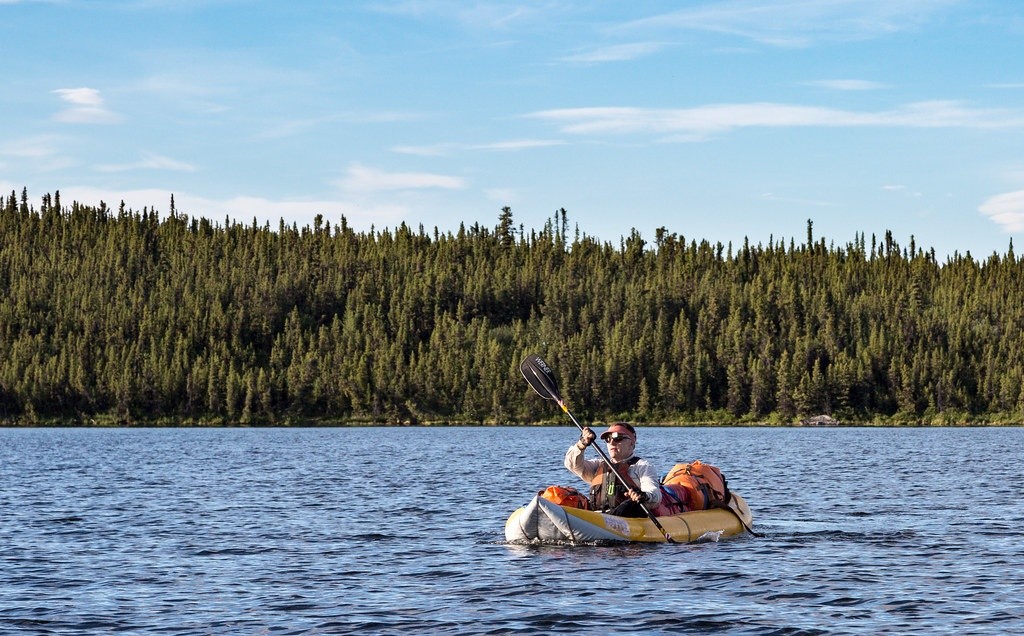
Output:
<box><xmin>505</xmin><ymin>493</ymin><xmax>752</xmax><ymax>545</ymax></box>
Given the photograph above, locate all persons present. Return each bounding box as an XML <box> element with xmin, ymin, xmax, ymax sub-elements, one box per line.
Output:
<box><xmin>564</xmin><ymin>423</ymin><xmax>662</xmax><ymax>518</ymax></box>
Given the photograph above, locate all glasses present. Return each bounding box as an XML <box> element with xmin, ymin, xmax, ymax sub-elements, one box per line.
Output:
<box><xmin>604</xmin><ymin>432</ymin><xmax>631</xmax><ymax>442</ymax></box>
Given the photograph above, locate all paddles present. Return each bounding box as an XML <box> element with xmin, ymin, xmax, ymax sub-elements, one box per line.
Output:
<box><xmin>519</xmin><ymin>352</ymin><xmax>679</xmax><ymax>545</ymax></box>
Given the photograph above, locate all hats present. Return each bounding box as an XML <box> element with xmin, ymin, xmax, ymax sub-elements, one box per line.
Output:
<box><xmin>600</xmin><ymin>425</ymin><xmax>635</xmax><ymax>442</ymax></box>
